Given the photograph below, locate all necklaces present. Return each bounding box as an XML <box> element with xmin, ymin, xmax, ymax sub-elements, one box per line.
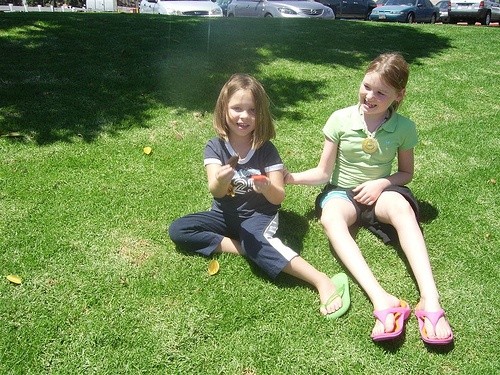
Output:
<box><xmin>360</xmin><ymin>111</ymin><xmax>389</xmax><ymax>154</ymax></box>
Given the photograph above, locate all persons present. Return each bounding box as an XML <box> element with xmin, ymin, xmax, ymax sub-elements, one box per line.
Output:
<box><xmin>169</xmin><ymin>73</ymin><xmax>350</xmax><ymax>319</ymax></box>
<box><xmin>283</xmin><ymin>53</ymin><xmax>454</xmax><ymax>344</ymax></box>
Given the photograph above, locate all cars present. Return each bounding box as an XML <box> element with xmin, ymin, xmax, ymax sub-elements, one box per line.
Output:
<box><xmin>139</xmin><ymin>0</ymin><xmax>223</xmax><ymax>18</ymax></box>
<box><xmin>321</xmin><ymin>0</ymin><xmax>378</xmax><ymax>21</ymax></box>
<box><xmin>436</xmin><ymin>0</ymin><xmax>500</xmax><ymax>25</ymax></box>
<box><xmin>227</xmin><ymin>0</ymin><xmax>336</xmax><ymax>21</ymax></box>
<box><xmin>369</xmin><ymin>0</ymin><xmax>442</xmax><ymax>24</ymax></box>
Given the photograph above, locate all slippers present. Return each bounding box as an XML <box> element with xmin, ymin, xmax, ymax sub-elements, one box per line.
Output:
<box><xmin>370</xmin><ymin>299</ymin><xmax>411</xmax><ymax>341</ymax></box>
<box><xmin>414</xmin><ymin>305</ymin><xmax>453</xmax><ymax>345</ymax></box>
<box><xmin>319</xmin><ymin>272</ymin><xmax>351</xmax><ymax>319</ymax></box>
